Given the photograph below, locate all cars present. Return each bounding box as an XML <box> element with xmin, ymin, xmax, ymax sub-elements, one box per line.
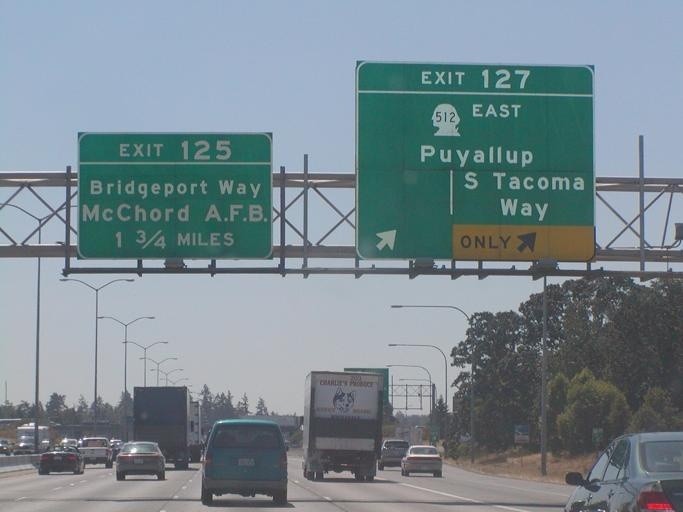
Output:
<box><xmin>562</xmin><ymin>430</ymin><xmax>682</xmax><ymax>511</ymax></box>
<box><xmin>401</xmin><ymin>445</ymin><xmax>442</xmax><ymax>479</ymax></box>
<box><xmin>0</xmin><ymin>423</ymin><xmax>167</xmax><ymax>483</ymax></box>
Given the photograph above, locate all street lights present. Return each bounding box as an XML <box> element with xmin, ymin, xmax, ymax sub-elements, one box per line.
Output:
<box><xmin>0</xmin><ymin>201</ymin><xmax>77</xmax><ymax>455</ymax></box>
<box><xmin>138</xmin><ymin>356</ymin><xmax>188</xmax><ymax>387</ymax></box>
<box><xmin>120</xmin><ymin>340</ymin><xmax>168</xmax><ymax>387</ymax></box>
<box><xmin>95</xmin><ymin>316</ymin><xmax>155</xmax><ymax>442</ymax></box>
<box><xmin>390</xmin><ymin>304</ymin><xmax>474</xmax><ymax>469</ymax></box>
<box><xmin>382</xmin><ymin>342</ymin><xmax>450</xmax><ymax>452</ymax></box>
<box><xmin>56</xmin><ymin>276</ymin><xmax>135</xmax><ymax>430</ymax></box>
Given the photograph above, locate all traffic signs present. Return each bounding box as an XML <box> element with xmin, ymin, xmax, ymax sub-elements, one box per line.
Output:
<box><xmin>74</xmin><ymin>132</ymin><xmax>276</xmax><ymax>264</ymax></box>
<box><xmin>354</xmin><ymin>57</ymin><xmax>597</xmax><ymax>265</ymax></box>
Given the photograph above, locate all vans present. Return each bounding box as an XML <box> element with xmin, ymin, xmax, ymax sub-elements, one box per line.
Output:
<box><xmin>198</xmin><ymin>418</ymin><xmax>287</xmax><ymax>506</ymax></box>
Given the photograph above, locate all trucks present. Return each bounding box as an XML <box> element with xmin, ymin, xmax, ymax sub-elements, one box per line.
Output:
<box><xmin>129</xmin><ymin>385</ymin><xmax>204</xmax><ymax>471</ymax></box>
<box><xmin>302</xmin><ymin>369</ymin><xmax>381</xmax><ymax>485</ymax></box>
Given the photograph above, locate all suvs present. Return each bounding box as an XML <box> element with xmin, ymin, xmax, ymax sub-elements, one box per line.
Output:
<box><xmin>378</xmin><ymin>439</ymin><xmax>410</xmax><ymax>471</ymax></box>
